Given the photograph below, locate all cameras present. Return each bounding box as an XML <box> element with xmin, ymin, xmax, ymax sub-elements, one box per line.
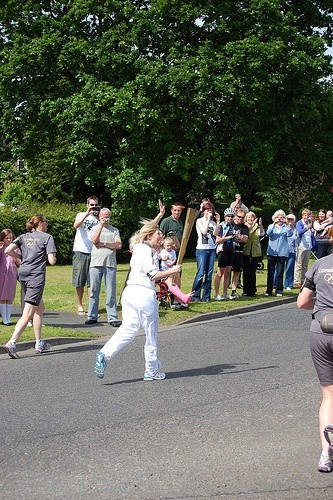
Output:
<box><xmin>93</xmin><ymin>207</ymin><xmax>100</xmax><ymax>212</ymax></box>
<box><xmin>232</xmin><ymin>234</ymin><xmax>236</xmax><ymax>239</ymax></box>
<box><xmin>211</xmin><ymin>213</ymin><xmax>216</xmax><ymax>217</ymax></box>
<box><xmin>103</xmin><ymin>217</ymin><xmax>110</xmax><ymax>222</ymax></box>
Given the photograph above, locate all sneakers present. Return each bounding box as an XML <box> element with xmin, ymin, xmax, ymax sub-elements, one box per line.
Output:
<box><xmin>3</xmin><ymin>342</ymin><xmax>20</xmax><ymax>359</ymax></box>
<box><xmin>143</xmin><ymin>371</ymin><xmax>166</xmax><ymax>380</ymax></box>
<box><xmin>318</xmin><ymin>455</ymin><xmax>333</xmax><ymax>471</ymax></box>
<box><xmin>35</xmin><ymin>340</ymin><xmax>51</xmax><ymax>353</ymax></box>
<box><xmin>323</xmin><ymin>424</ymin><xmax>333</xmax><ymax>461</ymax></box>
<box><xmin>94</xmin><ymin>352</ymin><xmax>108</xmax><ymax>379</ymax></box>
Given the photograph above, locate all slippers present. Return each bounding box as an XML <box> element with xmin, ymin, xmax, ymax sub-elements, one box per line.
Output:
<box><xmin>77</xmin><ymin>310</ymin><xmax>85</xmax><ymax>316</ymax></box>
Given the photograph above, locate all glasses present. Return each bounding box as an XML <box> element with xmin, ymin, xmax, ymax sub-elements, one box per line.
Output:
<box><xmin>227</xmin><ymin>216</ymin><xmax>234</xmax><ymax>218</ymax></box>
<box><xmin>90</xmin><ymin>203</ymin><xmax>98</xmax><ymax>206</ymax></box>
<box><xmin>237</xmin><ymin>215</ymin><xmax>245</xmax><ymax>219</ymax></box>
<box><xmin>42</xmin><ymin>220</ymin><xmax>48</xmax><ymax>223</ymax></box>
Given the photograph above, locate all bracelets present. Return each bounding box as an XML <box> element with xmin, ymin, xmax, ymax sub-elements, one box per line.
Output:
<box><xmin>103</xmin><ymin>242</ymin><xmax>106</xmax><ymax>248</ymax></box>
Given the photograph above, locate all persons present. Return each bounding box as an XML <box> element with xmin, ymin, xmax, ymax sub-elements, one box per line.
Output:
<box><xmin>94</xmin><ymin>199</ymin><xmax>182</xmax><ymax>381</ymax></box>
<box><xmin>296</xmin><ymin>226</ymin><xmax>333</xmax><ymax>473</ymax></box>
<box><xmin>0</xmin><ymin>228</ymin><xmax>22</xmax><ymax>325</ymax></box>
<box><xmin>72</xmin><ymin>196</ymin><xmax>101</xmax><ymax>318</ymax></box>
<box><xmin>3</xmin><ymin>215</ymin><xmax>57</xmax><ymax>359</ymax></box>
<box><xmin>85</xmin><ymin>208</ymin><xmax>123</xmax><ymax>327</ymax></box>
<box><xmin>156</xmin><ymin>193</ymin><xmax>333</xmax><ymax>310</ymax></box>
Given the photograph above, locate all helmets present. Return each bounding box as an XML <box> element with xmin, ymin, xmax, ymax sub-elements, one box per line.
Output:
<box><xmin>224</xmin><ymin>208</ymin><xmax>235</xmax><ymax>216</ymax></box>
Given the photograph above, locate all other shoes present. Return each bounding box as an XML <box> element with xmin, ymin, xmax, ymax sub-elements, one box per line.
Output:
<box><xmin>265</xmin><ymin>293</ymin><xmax>269</xmax><ymax>296</ymax></box>
<box><xmin>276</xmin><ymin>294</ymin><xmax>282</xmax><ymax>296</ymax></box>
<box><xmin>286</xmin><ymin>287</ymin><xmax>291</xmax><ymax>290</ymax></box>
<box><xmin>110</xmin><ymin>321</ymin><xmax>120</xmax><ymax>327</ymax></box>
<box><xmin>27</xmin><ymin>322</ymin><xmax>33</xmax><ymax>327</ymax></box>
<box><xmin>223</xmin><ymin>293</ymin><xmax>234</xmax><ymax>299</ymax></box>
<box><xmin>237</xmin><ymin>284</ymin><xmax>243</xmax><ymax>289</ymax></box>
<box><xmin>2</xmin><ymin>321</ymin><xmax>12</xmax><ymax>326</ymax></box>
<box><xmin>85</xmin><ymin>320</ymin><xmax>97</xmax><ymax>324</ymax></box>
<box><xmin>214</xmin><ymin>294</ymin><xmax>224</xmax><ymax>300</ymax></box>
<box><xmin>294</xmin><ymin>283</ymin><xmax>302</xmax><ymax>287</ymax></box>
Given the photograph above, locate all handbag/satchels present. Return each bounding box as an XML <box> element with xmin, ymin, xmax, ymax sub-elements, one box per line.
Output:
<box><xmin>310</xmin><ymin>228</ymin><xmax>318</xmax><ymax>250</ymax></box>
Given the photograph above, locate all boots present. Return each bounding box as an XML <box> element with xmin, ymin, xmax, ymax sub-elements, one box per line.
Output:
<box><xmin>168</xmin><ymin>283</ymin><xmax>192</xmax><ymax>304</ymax></box>
